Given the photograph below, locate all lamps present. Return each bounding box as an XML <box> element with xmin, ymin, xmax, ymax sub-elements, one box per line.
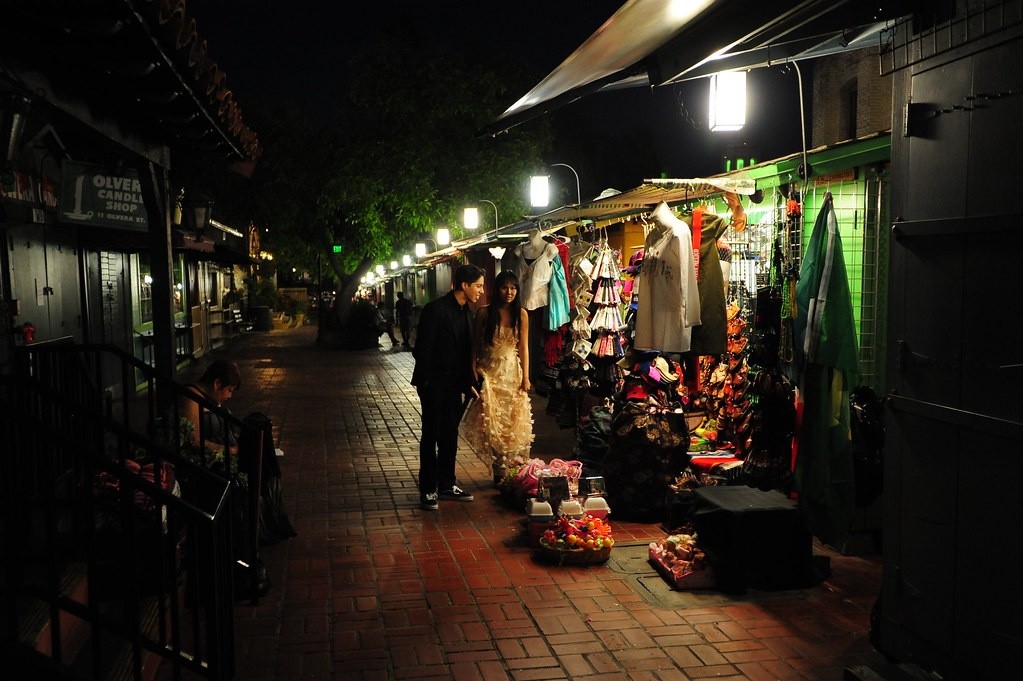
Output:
<box><xmin>529</xmin><ymin>163</ymin><xmax>582</xmax><ymax>209</ymax></box>
<box><xmin>708</xmin><ymin>56</ymin><xmax>812</xmax><ymax>186</ymax></box>
<box><xmin>363</xmin><ymin>199</ymin><xmax>500</xmax><ymax>286</ymax></box>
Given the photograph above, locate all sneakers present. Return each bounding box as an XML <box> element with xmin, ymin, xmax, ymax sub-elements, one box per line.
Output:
<box><xmin>420</xmin><ymin>491</ymin><xmax>439</xmax><ymax>510</ymax></box>
<box><xmin>437</xmin><ymin>484</ymin><xmax>474</xmax><ymax>502</ymax></box>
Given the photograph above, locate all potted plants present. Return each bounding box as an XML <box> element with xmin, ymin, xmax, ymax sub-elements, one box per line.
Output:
<box><xmin>249</xmin><ymin>275</ymin><xmax>277</xmax><ymax>332</ymax></box>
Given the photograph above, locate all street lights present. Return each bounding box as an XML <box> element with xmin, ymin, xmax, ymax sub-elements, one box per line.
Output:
<box><xmin>317</xmin><ymin>242</ymin><xmax>342</xmax><ymax>344</ymax></box>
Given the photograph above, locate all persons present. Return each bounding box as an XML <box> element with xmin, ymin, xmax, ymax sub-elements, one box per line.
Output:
<box><xmin>467</xmin><ymin>269</ymin><xmax>536</xmax><ymax>486</ymax></box>
<box><xmin>167</xmin><ymin>360</ymin><xmax>241</xmax><ymax>455</ymax></box>
<box><xmin>411</xmin><ymin>264</ymin><xmax>485</xmax><ymax>510</ymax></box>
<box><xmin>376</xmin><ymin>301</ymin><xmax>399</xmax><ymax>347</ymax></box>
<box><xmin>395</xmin><ymin>292</ymin><xmax>413</xmax><ymax>347</ymax></box>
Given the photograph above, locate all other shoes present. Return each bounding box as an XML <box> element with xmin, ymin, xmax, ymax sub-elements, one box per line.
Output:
<box><xmin>699</xmin><ymin>304</ymin><xmax>754</xmax><ymax>459</ymax></box>
<box><xmin>401</xmin><ymin>342</ymin><xmax>411</xmax><ymax>348</ymax></box>
<box><xmin>392</xmin><ymin>342</ymin><xmax>400</xmax><ymax>347</ymax></box>
<box><xmin>391</xmin><ymin>339</ymin><xmax>399</xmax><ymax>343</ymax></box>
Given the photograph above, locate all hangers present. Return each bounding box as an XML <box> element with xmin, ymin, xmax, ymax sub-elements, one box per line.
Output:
<box><xmin>519</xmin><ymin>217</ymin><xmax>572</xmax><ymax>254</ymax></box>
<box><xmin>675</xmin><ymin>201</ymin><xmax>727</xmax><ymax>233</ymax></box>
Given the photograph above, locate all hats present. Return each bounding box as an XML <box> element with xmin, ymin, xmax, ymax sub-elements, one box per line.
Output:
<box><xmin>616</xmin><ymin>248</ymin><xmax>680</xmax><ymax>403</ymax></box>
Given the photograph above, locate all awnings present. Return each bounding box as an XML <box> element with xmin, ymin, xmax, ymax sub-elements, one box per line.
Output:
<box><xmin>420</xmin><ymin>176</ymin><xmax>754</xmax><ymax>265</ymax></box>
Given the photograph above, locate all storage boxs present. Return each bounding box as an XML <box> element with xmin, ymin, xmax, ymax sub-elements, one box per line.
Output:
<box><xmin>526</xmin><ymin>494</ymin><xmax>612</xmax><ymax>550</ymax></box>
